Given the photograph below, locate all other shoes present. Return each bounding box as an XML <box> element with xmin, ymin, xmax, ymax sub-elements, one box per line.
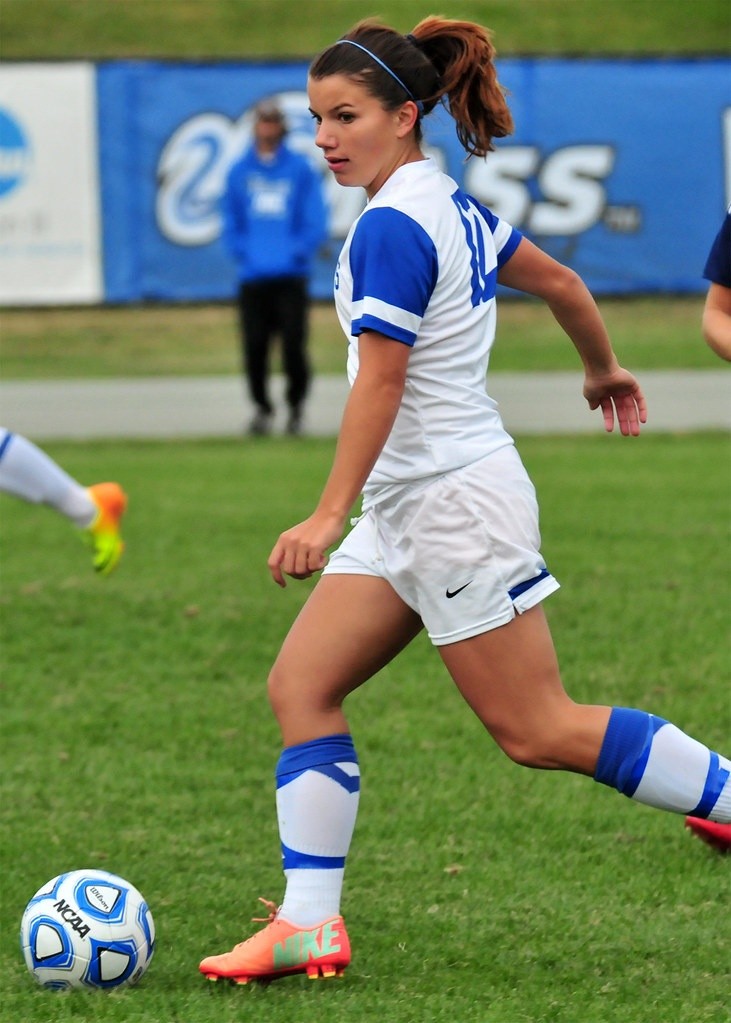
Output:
<box><xmin>250</xmin><ymin>409</ymin><xmax>306</xmax><ymax>439</ymax></box>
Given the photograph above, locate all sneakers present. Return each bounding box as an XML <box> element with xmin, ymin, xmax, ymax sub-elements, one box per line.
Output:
<box><xmin>197</xmin><ymin>917</ymin><xmax>351</xmax><ymax>984</ymax></box>
<box><xmin>84</xmin><ymin>481</ymin><xmax>126</xmax><ymax>574</ymax></box>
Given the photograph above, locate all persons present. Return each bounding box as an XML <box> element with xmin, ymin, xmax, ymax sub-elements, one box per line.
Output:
<box><xmin>194</xmin><ymin>12</ymin><xmax>730</xmax><ymax>991</ymax></box>
<box><xmin>701</xmin><ymin>200</ymin><xmax>731</xmax><ymax>367</ymax></box>
<box><xmin>0</xmin><ymin>424</ymin><xmax>129</xmax><ymax>581</ymax></box>
<box><xmin>216</xmin><ymin>94</ymin><xmax>332</xmax><ymax>439</ymax></box>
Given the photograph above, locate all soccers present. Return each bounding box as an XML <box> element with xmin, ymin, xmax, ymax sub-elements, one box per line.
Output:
<box><xmin>20</xmin><ymin>869</ymin><xmax>158</xmax><ymax>994</ymax></box>
